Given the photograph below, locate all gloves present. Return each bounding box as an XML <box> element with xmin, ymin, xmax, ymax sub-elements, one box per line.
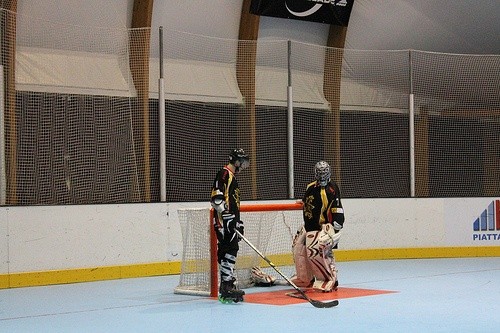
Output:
<box><xmin>221</xmin><ymin>210</ymin><xmax>236</xmax><ymax>232</ymax></box>
<box><xmin>235</xmin><ymin>220</ymin><xmax>244</xmax><ymax>242</ymax></box>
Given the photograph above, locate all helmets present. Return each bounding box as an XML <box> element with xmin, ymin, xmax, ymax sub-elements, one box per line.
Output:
<box><xmin>229</xmin><ymin>148</ymin><xmax>250</xmax><ymax>169</ymax></box>
<box><xmin>315</xmin><ymin>160</ymin><xmax>331</xmax><ymax>186</ymax></box>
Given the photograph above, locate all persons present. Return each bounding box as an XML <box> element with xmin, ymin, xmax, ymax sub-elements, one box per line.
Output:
<box><xmin>210</xmin><ymin>148</ymin><xmax>250</xmax><ymax>303</ymax></box>
<box><xmin>286</xmin><ymin>161</ymin><xmax>345</xmax><ymax>292</ymax></box>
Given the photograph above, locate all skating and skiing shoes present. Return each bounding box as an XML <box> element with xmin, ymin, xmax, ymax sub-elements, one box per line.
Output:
<box><xmin>219</xmin><ymin>277</ymin><xmax>245</xmax><ymax>303</ymax></box>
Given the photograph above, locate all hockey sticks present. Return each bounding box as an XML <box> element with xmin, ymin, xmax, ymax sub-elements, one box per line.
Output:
<box><xmin>233</xmin><ymin>227</ymin><xmax>339</xmax><ymax>307</ymax></box>
